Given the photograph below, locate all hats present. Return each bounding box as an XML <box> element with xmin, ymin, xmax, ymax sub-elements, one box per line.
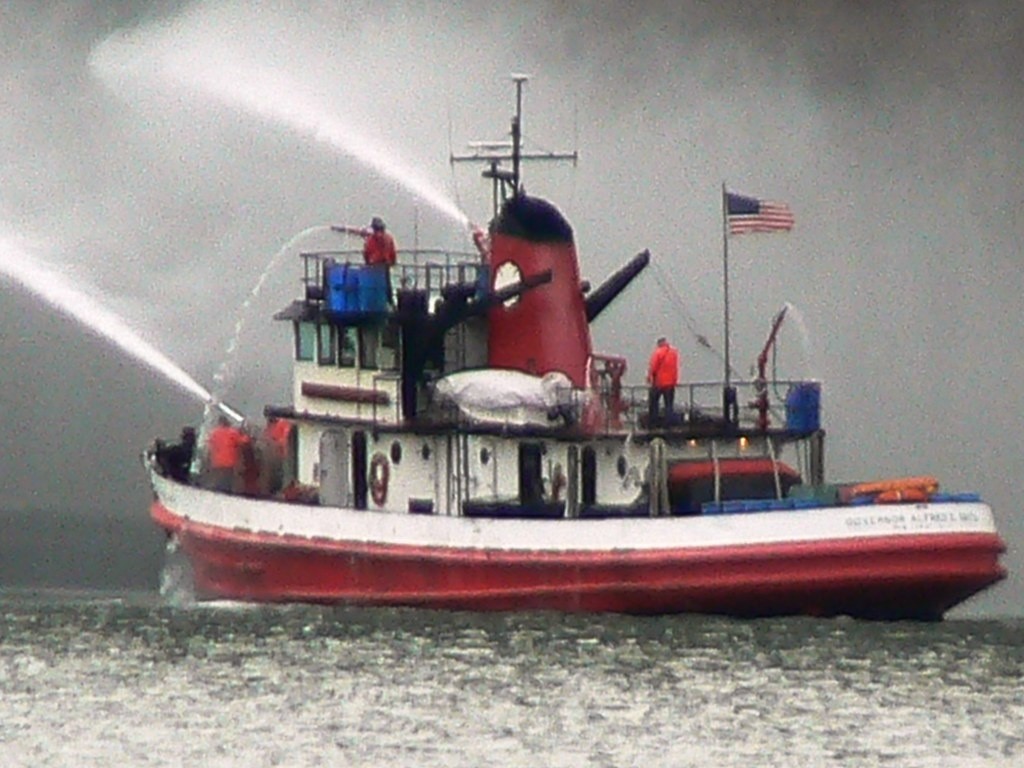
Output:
<box><xmin>657</xmin><ymin>336</ymin><xmax>666</xmax><ymax>345</ymax></box>
<box><xmin>371</xmin><ymin>217</ymin><xmax>385</xmax><ymax>228</ymax></box>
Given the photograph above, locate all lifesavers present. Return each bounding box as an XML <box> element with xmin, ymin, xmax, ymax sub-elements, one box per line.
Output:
<box><xmin>878</xmin><ymin>488</ymin><xmax>924</xmax><ymax>503</ymax></box>
<box><xmin>369</xmin><ymin>452</ymin><xmax>389</xmax><ymax>506</ymax></box>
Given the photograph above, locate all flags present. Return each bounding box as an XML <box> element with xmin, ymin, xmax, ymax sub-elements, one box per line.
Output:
<box><xmin>728</xmin><ymin>191</ymin><xmax>795</xmax><ymax>234</ymax></box>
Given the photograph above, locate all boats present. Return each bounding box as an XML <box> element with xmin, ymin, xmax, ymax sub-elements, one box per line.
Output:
<box><xmin>140</xmin><ymin>75</ymin><xmax>1007</xmax><ymax>620</ymax></box>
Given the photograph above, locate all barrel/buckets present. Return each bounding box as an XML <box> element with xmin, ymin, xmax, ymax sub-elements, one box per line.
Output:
<box><xmin>784</xmin><ymin>383</ymin><xmax>819</xmax><ymax>431</ymax></box>
<box><xmin>358</xmin><ymin>266</ymin><xmax>389</xmax><ymax>314</ymax></box>
<box><xmin>326</xmin><ymin>265</ymin><xmax>358</xmax><ymax>313</ymax></box>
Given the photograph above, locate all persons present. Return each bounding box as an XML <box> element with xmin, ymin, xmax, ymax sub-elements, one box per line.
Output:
<box><xmin>264</xmin><ymin>413</ymin><xmax>292</xmax><ymax>490</ymax></box>
<box><xmin>208</xmin><ymin>416</ymin><xmax>249</xmax><ymax>494</ymax></box>
<box><xmin>360</xmin><ymin>217</ymin><xmax>397</xmax><ymax>312</ymax></box>
<box><xmin>647</xmin><ymin>336</ymin><xmax>679</xmax><ymax>429</ymax></box>
